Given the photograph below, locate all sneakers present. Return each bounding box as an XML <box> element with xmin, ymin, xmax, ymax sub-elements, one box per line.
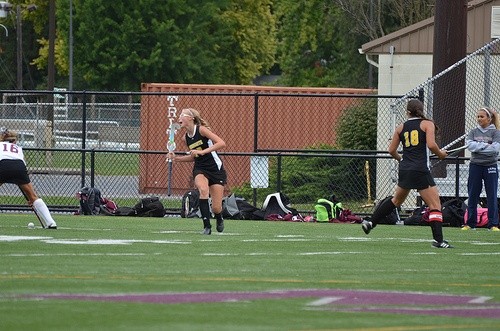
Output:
<box><xmin>362</xmin><ymin>217</ymin><xmax>373</xmax><ymax>234</ymax></box>
<box><xmin>200</xmin><ymin>225</ymin><xmax>211</xmax><ymax>235</ymax></box>
<box><xmin>216</xmin><ymin>216</ymin><xmax>224</xmax><ymax>232</ymax></box>
<box><xmin>431</xmin><ymin>239</ymin><xmax>454</xmax><ymax>248</ymax></box>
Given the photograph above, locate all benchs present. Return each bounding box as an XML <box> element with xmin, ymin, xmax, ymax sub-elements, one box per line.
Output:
<box><xmin>417</xmin><ymin>163</ymin><xmax>500</xmax><ymax>207</ymax></box>
<box><xmin>27</xmin><ymin>165</ymin><xmax>90</xmax><ymax>188</ymax></box>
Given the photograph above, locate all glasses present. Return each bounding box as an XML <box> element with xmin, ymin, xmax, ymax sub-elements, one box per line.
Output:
<box><xmin>180</xmin><ymin>114</ymin><xmax>192</xmax><ymax>118</ymax></box>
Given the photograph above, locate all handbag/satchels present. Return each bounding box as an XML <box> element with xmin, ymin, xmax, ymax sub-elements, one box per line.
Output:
<box><xmin>463</xmin><ymin>207</ymin><xmax>489</xmax><ymax>226</ymax></box>
<box><xmin>74</xmin><ymin>187</ymin><xmax>166</xmax><ymax>217</ymax></box>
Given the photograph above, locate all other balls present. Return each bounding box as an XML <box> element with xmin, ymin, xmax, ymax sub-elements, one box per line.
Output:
<box><xmin>27</xmin><ymin>222</ymin><xmax>35</xmax><ymax>228</ymax></box>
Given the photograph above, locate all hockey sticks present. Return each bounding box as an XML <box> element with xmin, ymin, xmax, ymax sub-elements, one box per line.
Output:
<box><xmin>401</xmin><ymin>145</ymin><xmax>469</xmax><ymax>160</ymax></box>
<box><xmin>16</xmin><ymin>185</ymin><xmax>45</xmax><ymax>229</ymax></box>
<box><xmin>166</xmin><ymin>122</ymin><xmax>181</xmax><ymax>196</ymax></box>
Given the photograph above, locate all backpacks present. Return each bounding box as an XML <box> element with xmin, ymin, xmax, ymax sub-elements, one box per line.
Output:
<box><xmin>261</xmin><ymin>192</ymin><xmax>299</xmax><ymax>217</ymax></box>
<box><xmin>314</xmin><ymin>195</ymin><xmax>345</xmax><ymax>222</ymax></box>
<box><xmin>404</xmin><ymin>199</ymin><xmax>467</xmax><ymax>227</ymax></box>
<box><xmin>375</xmin><ymin>196</ymin><xmax>399</xmax><ymax>224</ymax></box>
<box><xmin>181</xmin><ymin>191</ymin><xmax>200</xmax><ymax>218</ymax></box>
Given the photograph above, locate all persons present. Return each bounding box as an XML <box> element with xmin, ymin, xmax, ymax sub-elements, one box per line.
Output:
<box><xmin>461</xmin><ymin>107</ymin><xmax>500</xmax><ymax>232</ymax></box>
<box><xmin>0</xmin><ymin>129</ymin><xmax>57</xmax><ymax>230</ymax></box>
<box><xmin>166</xmin><ymin>109</ymin><xmax>227</xmax><ymax>235</ymax></box>
<box><xmin>362</xmin><ymin>100</ymin><xmax>453</xmax><ymax>249</ymax></box>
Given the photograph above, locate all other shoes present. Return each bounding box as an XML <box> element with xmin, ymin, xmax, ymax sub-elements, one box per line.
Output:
<box><xmin>490</xmin><ymin>226</ymin><xmax>500</xmax><ymax>232</ymax></box>
<box><xmin>462</xmin><ymin>225</ymin><xmax>472</xmax><ymax>231</ymax></box>
<box><xmin>45</xmin><ymin>224</ymin><xmax>57</xmax><ymax>229</ymax></box>
